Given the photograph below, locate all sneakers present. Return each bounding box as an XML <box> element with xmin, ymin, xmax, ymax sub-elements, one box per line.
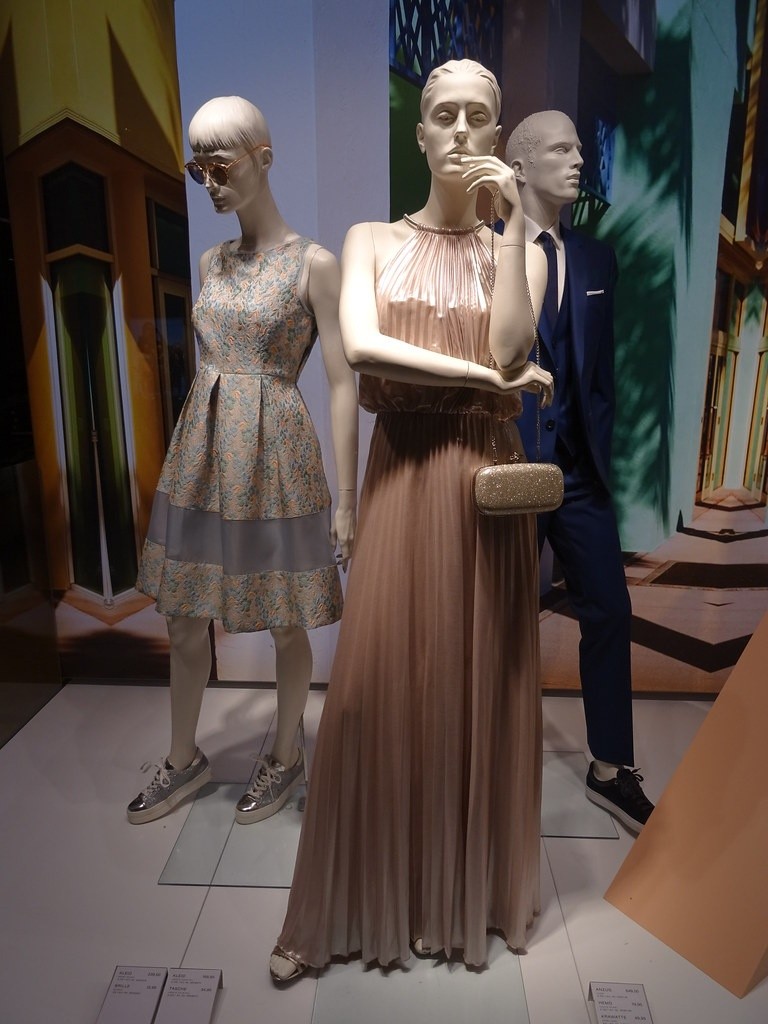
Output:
<box><xmin>234</xmin><ymin>747</ymin><xmax>304</xmax><ymax>825</ymax></box>
<box><xmin>585</xmin><ymin>761</ymin><xmax>655</xmax><ymax>832</ymax></box>
<box><xmin>127</xmin><ymin>745</ymin><xmax>214</xmax><ymax>825</ymax></box>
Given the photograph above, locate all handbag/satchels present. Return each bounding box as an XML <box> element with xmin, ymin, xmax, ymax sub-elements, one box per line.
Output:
<box><xmin>474</xmin><ymin>460</ymin><xmax>563</xmax><ymax>517</ymax></box>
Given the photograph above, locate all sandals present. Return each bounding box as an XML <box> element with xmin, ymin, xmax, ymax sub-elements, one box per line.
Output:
<box><xmin>408</xmin><ymin>936</ymin><xmax>441</xmax><ymax>959</ymax></box>
<box><xmin>269</xmin><ymin>945</ymin><xmax>310</xmax><ymax>980</ymax></box>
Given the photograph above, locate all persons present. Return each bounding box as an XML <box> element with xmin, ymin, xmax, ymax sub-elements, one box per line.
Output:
<box><xmin>484</xmin><ymin>110</ymin><xmax>656</xmax><ymax>833</ymax></box>
<box><xmin>267</xmin><ymin>59</ymin><xmax>554</xmax><ymax>987</ymax></box>
<box><xmin>123</xmin><ymin>95</ymin><xmax>360</xmax><ymax>827</ymax></box>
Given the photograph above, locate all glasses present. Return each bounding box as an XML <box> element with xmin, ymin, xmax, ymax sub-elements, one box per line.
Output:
<box><xmin>183</xmin><ymin>143</ymin><xmax>271</xmax><ymax>188</ymax></box>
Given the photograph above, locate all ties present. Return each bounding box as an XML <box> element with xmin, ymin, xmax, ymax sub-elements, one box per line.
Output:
<box><xmin>537</xmin><ymin>232</ymin><xmax>558</xmax><ymax>338</ymax></box>
